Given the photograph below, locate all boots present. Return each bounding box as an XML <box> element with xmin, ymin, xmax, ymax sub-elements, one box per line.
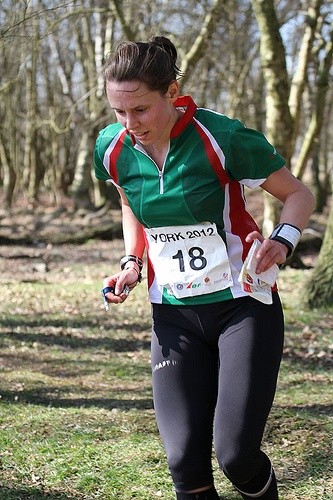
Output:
<box><xmin>231</xmin><ymin>465</ymin><xmax>278</xmax><ymax>500</ymax></box>
<box><xmin>169</xmin><ymin>486</ymin><xmax>221</xmax><ymax>500</ymax></box>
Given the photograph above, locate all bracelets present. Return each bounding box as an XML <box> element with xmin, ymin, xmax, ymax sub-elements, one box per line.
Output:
<box><xmin>120</xmin><ymin>255</ymin><xmax>143</xmax><ymax>276</ymax></box>
<box><xmin>269</xmin><ymin>223</ymin><xmax>302</xmax><ymax>258</ymax></box>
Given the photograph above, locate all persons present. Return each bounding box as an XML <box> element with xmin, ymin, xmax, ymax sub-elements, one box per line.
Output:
<box><xmin>93</xmin><ymin>36</ymin><xmax>316</xmax><ymax>500</ymax></box>
